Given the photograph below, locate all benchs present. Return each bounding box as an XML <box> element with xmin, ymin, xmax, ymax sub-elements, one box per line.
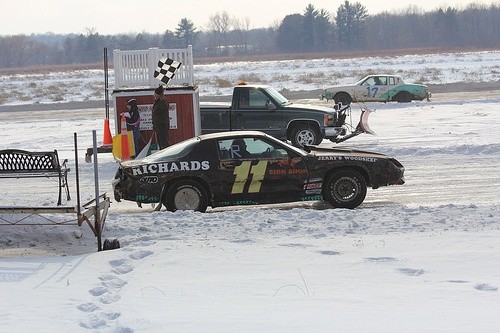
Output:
<box><xmin>0</xmin><ymin>149</ymin><xmax>71</xmax><ymax>206</ymax></box>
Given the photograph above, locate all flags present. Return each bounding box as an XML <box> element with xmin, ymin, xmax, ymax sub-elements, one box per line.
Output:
<box><xmin>154</xmin><ymin>57</ymin><xmax>181</xmax><ymax>84</ymax></box>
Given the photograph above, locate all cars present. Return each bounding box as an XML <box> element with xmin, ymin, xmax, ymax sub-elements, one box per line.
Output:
<box><xmin>321</xmin><ymin>74</ymin><xmax>432</xmax><ymax>105</ymax></box>
<box><xmin>115</xmin><ymin>130</ymin><xmax>406</xmax><ymax>210</ymax></box>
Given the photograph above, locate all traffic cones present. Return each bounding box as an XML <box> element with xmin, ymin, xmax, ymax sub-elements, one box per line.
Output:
<box><xmin>102</xmin><ymin>117</ymin><xmax>114</xmax><ymax>149</ymax></box>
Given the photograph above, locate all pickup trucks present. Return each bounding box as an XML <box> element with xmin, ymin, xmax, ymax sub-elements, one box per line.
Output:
<box><xmin>199</xmin><ymin>82</ymin><xmax>351</xmax><ymax>154</ymax></box>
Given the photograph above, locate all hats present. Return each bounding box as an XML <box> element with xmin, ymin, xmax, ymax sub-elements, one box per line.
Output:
<box><xmin>128</xmin><ymin>99</ymin><xmax>137</xmax><ymax>108</ymax></box>
<box><xmin>155</xmin><ymin>86</ymin><xmax>164</xmax><ymax>95</ymax></box>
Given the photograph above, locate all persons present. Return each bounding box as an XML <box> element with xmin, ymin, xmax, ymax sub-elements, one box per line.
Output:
<box><xmin>120</xmin><ymin>99</ymin><xmax>140</xmax><ymax>159</ymax></box>
<box><xmin>373</xmin><ymin>77</ymin><xmax>379</xmax><ymax>85</ymax></box>
<box><xmin>152</xmin><ymin>85</ymin><xmax>170</xmax><ymax>150</ymax></box>
<box><xmin>230</xmin><ymin>139</ymin><xmax>252</xmax><ymax>159</ymax></box>
